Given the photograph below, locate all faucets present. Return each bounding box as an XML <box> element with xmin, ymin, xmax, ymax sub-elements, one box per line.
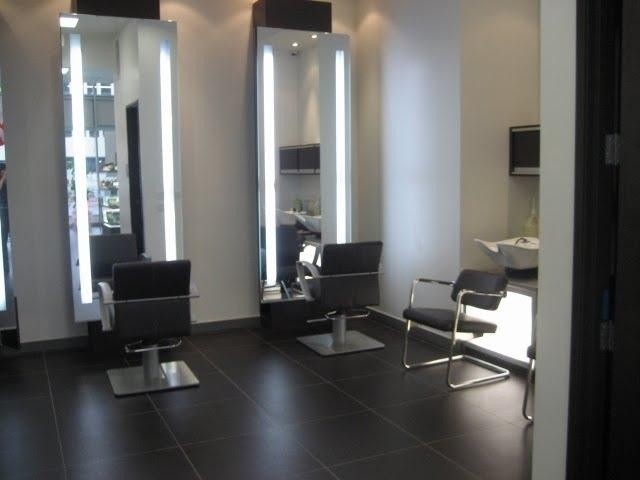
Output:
<box><xmin>515</xmin><ymin>238</ymin><xmax>528</xmax><ymax>246</ymax></box>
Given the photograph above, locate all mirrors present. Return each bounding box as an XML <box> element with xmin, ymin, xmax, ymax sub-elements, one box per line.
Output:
<box><xmin>1</xmin><ymin>80</ymin><xmax>19</xmax><ymax>333</ymax></box>
<box><xmin>58</xmin><ymin>11</ymin><xmax>186</xmax><ymax>324</ymax></box>
<box><xmin>256</xmin><ymin>25</ymin><xmax>353</xmax><ymax>305</ymax></box>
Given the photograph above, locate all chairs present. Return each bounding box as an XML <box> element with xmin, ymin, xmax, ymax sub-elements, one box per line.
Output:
<box><xmin>403</xmin><ymin>267</ymin><xmax>510</xmax><ymax>390</ymax></box>
<box><xmin>96</xmin><ymin>258</ymin><xmax>201</xmax><ymax>397</ymax></box>
<box><xmin>295</xmin><ymin>240</ymin><xmax>387</xmax><ymax>357</ymax></box>
<box><xmin>258</xmin><ymin>225</ymin><xmax>301</xmax><ymax>299</ymax></box>
<box><xmin>522</xmin><ymin>312</ymin><xmax>536</xmax><ymax>422</ymax></box>
<box><xmin>84</xmin><ymin>233</ymin><xmax>138</xmax><ymax>291</ymax></box>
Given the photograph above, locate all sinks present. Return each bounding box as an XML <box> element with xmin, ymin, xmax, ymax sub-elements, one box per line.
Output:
<box><xmin>473</xmin><ymin>237</ymin><xmax>540</xmax><ymax>271</ymax></box>
<box><xmin>295</xmin><ymin>214</ymin><xmax>321</xmax><ymax>235</ymax></box>
<box><xmin>276</xmin><ymin>208</ymin><xmax>307</xmax><ymax>226</ymax></box>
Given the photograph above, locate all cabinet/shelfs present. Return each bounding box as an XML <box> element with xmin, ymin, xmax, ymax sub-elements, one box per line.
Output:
<box><xmin>279</xmin><ymin>143</ymin><xmax>321</xmax><ymax>175</ymax></box>
<box><xmin>508</xmin><ymin>124</ymin><xmax>541</xmax><ymax>177</ymax></box>
